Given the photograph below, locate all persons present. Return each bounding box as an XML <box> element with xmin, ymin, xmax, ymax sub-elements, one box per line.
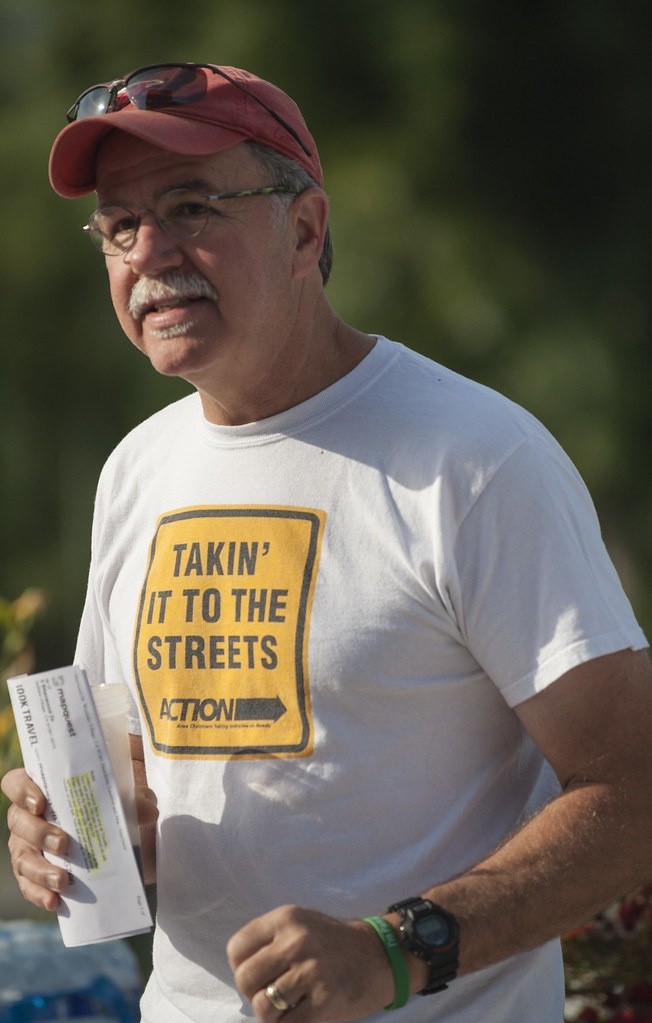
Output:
<box><xmin>0</xmin><ymin>61</ymin><xmax>650</xmax><ymax>1023</ymax></box>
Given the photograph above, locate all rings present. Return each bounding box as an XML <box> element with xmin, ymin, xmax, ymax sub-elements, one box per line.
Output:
<box><xmin>266</xmin><ymin>983</ymin><xmax>293</xmax><ymax>1014</ymax></box>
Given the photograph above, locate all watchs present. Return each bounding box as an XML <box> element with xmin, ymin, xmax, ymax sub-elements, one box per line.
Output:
<box><xmin>387</xmin><ymin>896</ymin><xmax>462</xmax><ymax>997</ymax></box>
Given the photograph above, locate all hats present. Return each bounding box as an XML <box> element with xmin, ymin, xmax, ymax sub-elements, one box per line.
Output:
<box><xmin>50</xmin><ymin>63</ymin><xmax>323</xmax><ymax>199</ymax></box>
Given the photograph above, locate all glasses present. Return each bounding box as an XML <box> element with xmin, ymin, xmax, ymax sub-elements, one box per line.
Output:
<box><xmin>65</xmin><ymin>63</ymin><xmax>313</xmax><ymax>161</ymax></box>
<box><xmin>81</xmin><ymin>187</ymin><xmax>301</xmax><ymax>254</ymax></box>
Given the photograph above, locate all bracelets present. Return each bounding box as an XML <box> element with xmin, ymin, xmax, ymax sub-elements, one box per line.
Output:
<box><xmin>360</xmin><ymin>914</ymin><xmax>411</xmax><ymax>1010</ymax></box>
